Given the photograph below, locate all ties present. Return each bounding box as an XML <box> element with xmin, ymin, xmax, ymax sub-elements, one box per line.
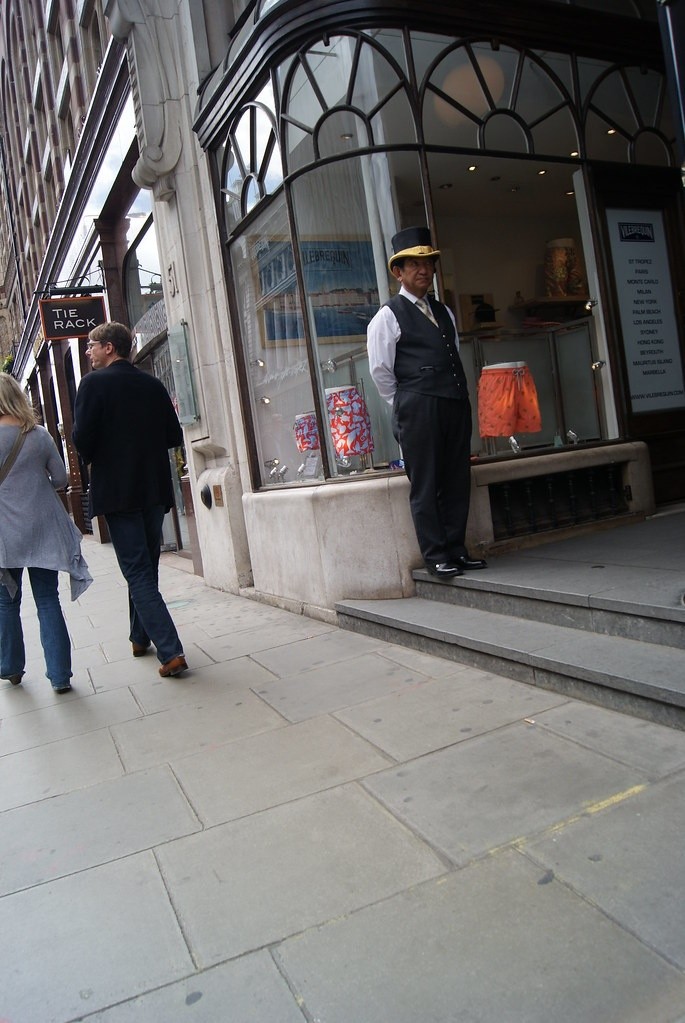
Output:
<box><xmin>416</xmin><ymin>298</ymin><xmax>439</xmax><ymax>328</ymax></box>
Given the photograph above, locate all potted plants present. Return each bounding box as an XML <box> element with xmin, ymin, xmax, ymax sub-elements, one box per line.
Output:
<box><xmin>2</xmin><ymin>355</ymin><xmax>14</xmax><ymax>373</ymax></box>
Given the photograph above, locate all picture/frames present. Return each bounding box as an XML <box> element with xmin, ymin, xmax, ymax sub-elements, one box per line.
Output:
<box><xmin>244</xmin><ymin>234</ymin><xmax>380</xmax><ymax>350</ymax></box>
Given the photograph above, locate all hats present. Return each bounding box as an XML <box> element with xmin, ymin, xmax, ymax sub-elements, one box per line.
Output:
<box><xmin>387</xmin><ymin>226</ymin><xmax>441</xmax><ymax>280</ymax></box>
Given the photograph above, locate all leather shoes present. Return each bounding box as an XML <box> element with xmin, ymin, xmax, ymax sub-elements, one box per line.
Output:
<box><xmin>425</xmin><ymin>562</ymin><xmax>462</xmax><ymax>578</ymax></box>
<box><xmin>133</xmin><ymin>641</ymin><xmax>146</xmax><ymax>657</ymax></box>
<box><xmin>159</xmin><ymin>654</ymin><xmax>188</xmax><ymax>677</ymax></box>
<box><xmin>449</xmin><ymin>555</ymin><xmax>487</xmax><ymax>569</ymax></box>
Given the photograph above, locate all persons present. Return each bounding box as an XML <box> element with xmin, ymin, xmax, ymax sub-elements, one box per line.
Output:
<box><xmin>0</xmin><ymin>372</ymin><xmax>94</xmax><ymax>691</ymax></box>
<box><xmin>367</xmin><ymin>225</ymin><xmax>486</xmax><ymax>579</ymax></box>
<box><xmin>71</xmin><ymin>322</ymin><xmax>189</xmax><ymax>676</ymax></box>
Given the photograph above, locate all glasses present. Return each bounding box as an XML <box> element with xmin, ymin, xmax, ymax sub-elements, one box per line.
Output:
<box><xmin>87</xmin><ymin>341</ymin><xmax>113</xmax><ymax>349</ymax></box>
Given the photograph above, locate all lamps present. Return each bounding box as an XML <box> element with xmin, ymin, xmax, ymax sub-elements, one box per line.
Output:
<box><xmin>251</xmin><ymin>359</ymin><xmax>264</xmax><ymax>367</ymax></box>
<box><xmin>264</xmin><ymin>459</ymin><xmax>306</xmax><ymax>483</ymax></box>
<box><xmin>567</xmin><ymin>430</ymin><xmax>581</xmax><ymax>445</ymax></box>
<box><xmin>508</xmin><ymin>435</ymin><xmax>521</xmax><ymax>454</ymax></box>
<box><xmin>585</xmin><ymin>300</ymin><xmax>599</xmax><ymax>310</ymax></box>
<box><xmin>592</xmin><ymin>360</ymin><xmax>604</xmax><ymax>371</ymax></box>
<box><xmin>260</xmin><ymin>396</ymin><xmax>271</xmax><ymax>404</ymax></box>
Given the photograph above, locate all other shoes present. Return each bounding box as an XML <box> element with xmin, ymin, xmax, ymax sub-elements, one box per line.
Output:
<box><xmin>9</xmin><ymin>674</ymin><xmax>21</xmax><ymax>685</ymax></box>
<box><xmin>52</xmin><ymin>687</ymin><xmax>69</xmax><ymax>693</ymax></box>
